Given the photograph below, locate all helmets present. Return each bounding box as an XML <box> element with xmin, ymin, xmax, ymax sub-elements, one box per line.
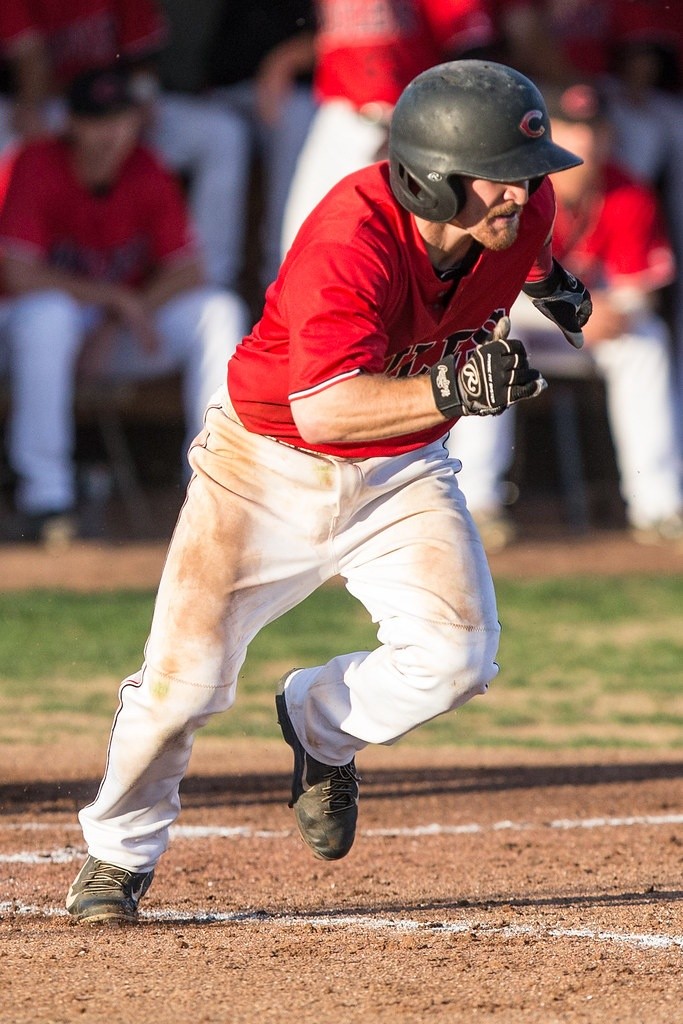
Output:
<box><xmin>388</xmin><ymin>59</ymin><xmax>584</xmax><ymax>222</ymax></box>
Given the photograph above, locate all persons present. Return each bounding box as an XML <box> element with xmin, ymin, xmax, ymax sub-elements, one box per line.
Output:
<box><xmin>2</xmin><ymin>69</ymin><xmax>251</xmax><ymax>546</ymax></box>
<box><xmin>0</xmin><ymin>0</ymin><xmax>682</xmax><ymax>553</ymax></box>
<box><xmin>64</xmin><ymin>60</ymin><xmax>592</xmax><ymax>924</ymax></box>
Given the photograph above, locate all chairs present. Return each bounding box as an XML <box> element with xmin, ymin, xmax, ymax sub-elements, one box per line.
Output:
<box><xmin>0</xmin><ymin>93</ymin><xmax>264</xmax><ymax>545</ymax></box>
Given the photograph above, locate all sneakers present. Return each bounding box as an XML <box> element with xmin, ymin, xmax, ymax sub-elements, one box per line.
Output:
<box><xmin>65</xmin><ymin>855</ymin><xmax>154</xmax><ymax>923</ymax></box>
<box><xmin>275</xmin><ymin>668</ymin><xmax>362</xmax><ymax>860</ymax></box>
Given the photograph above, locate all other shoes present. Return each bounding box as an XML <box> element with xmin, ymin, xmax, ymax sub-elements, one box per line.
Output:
<box><xmin>40</xmin><ymin>511</ymin><xmax>80</xmax><ymax>560</ymax></box>
<box><xmin>472</xmin><ymin>508</ymin><xmax>517</xmax><ymax>553</ymax></box>
<box><xmin>628</xmin><ymin>515</ymin><xmax>683</xmax><ymax>544</ymax></box>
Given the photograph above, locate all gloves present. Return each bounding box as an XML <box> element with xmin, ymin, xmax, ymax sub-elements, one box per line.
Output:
<box><xmin>430</xmin><ymin>316</ymin><xmax>549</xmax><ymax>420</ymax></box>
<box><xmin>521</xmin><ymin>256</ymin><xmax>593</xmax><ymax>349</ymax></box>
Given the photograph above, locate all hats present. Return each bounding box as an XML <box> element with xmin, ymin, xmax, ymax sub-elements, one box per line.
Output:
<box><xmin>65</xmin><ymin>66</ymin><xmax>153</xmax><ymax>116</ymax></box>
<box><xmin>550</xmin><ymin>78</ymin><xmax>611</xmax><ymax>125</ymax></box>
